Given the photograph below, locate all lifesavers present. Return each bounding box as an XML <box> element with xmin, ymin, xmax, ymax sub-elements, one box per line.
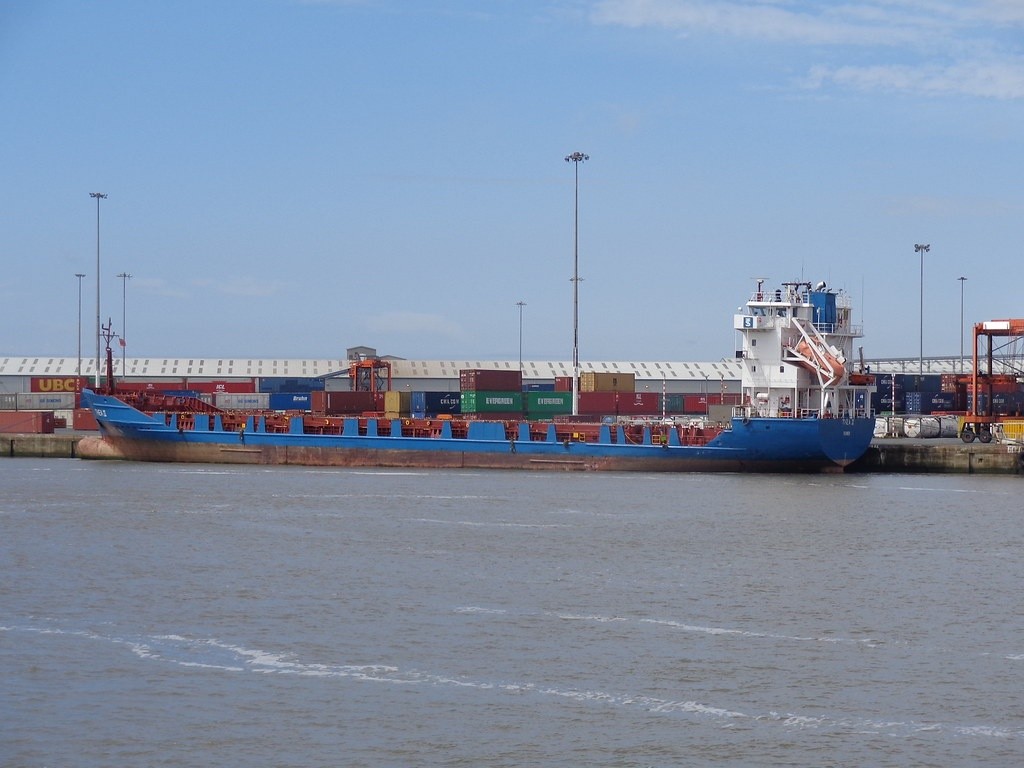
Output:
<box><xmin>465</xmin><ymin>424</ymin><xmax>469</xmax><ymax>428</ymax></box>
<box><xmin>180</xmin><ymin>415</ymin><xmax>185</xmax><ymax>419</ymax></box>
<box><xmin>426</xmin><ymin>421</ymin><xmax>430</xmax><ymax>426</ymax></box>
<box><xmin>405</xmin><ymin>420</ymin><xmax>410</xmax><ymax>425</ymax></box>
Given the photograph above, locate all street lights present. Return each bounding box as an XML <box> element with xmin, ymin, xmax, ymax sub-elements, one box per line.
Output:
<box><xmin>514</xmin><ymin>301</ymin><xmax>528</xmax><ymax>371</ymax></box>
<box><xmin>89</xmin><ymin>192</ymin><xmax>109</xmax><ymax>389</ymax></box>
<box><xmin>913</xmin><ymin>243</ymin><xmax>931</xmax><ymax>376</ymax></box>
<box><xmin>564</xmin><ymin>151</ymin><xmax>590</xmax><ymax>415</ymax></box>
<box><xmin>116</xmin><ymin>272</ymin><xmax>133</xmax><ymax>379</ymax></box>
<box><xmin>75</xmin><ymin>273</ymin><xmax>86</xmax><ymax>377</ymax></box>
<box><xmin>956</xmin><ymin>276</ymin><xmax>968</xmax><ymax>374</ymax></box>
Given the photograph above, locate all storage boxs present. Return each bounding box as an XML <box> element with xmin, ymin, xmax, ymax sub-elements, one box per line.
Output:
<box><xmin>0</xmin><ymin>369</ymin><xmax>744</xmax><ymax>434</ymax></box>
<box><xmin>851</xmin><ymin>374</ymin><xmax>1024</xmax><ymax>439</ymax></box>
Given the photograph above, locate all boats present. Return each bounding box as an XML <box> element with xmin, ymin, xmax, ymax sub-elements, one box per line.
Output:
<box><xmin>76</xmin><ymin>275</ymin><xmax>878</xmax><ymax>475</ymax></box>
